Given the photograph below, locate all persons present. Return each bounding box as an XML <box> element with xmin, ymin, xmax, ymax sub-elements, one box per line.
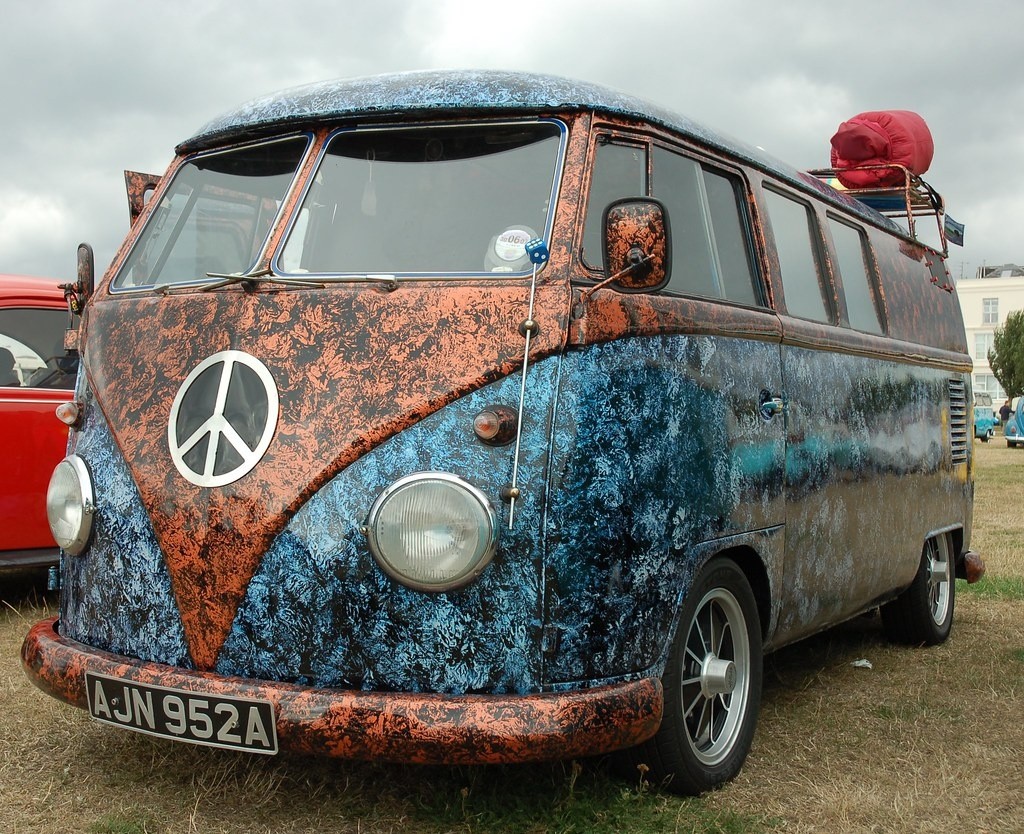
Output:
<box><xmin>1000</xmin><ymin>401</ymin><xmax>1015</xmax><ymax>435</ymax></box>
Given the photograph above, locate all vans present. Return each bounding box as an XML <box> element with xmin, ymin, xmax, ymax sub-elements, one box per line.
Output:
<box><xmin>972</xmin><ymin>391</ymin><xmax>995</xmax><ymax>443</ymax></box>
<box><xmin>20</xmin><ymin>63</ymin><xmax>986</xmax><ymax>792</ymax></box>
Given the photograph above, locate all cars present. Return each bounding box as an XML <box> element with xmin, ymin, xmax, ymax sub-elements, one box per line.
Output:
<box><xmin>1004</xmin><ymin>396</ymin><xmax>1024</xmax><ymax>447</ymax></box>
<box><xmin>0</xmin><ymin>276</ymin><xmax>96</xmax><ymax>575</ymax></box>
<box><xmin>993</xmin><ymin>417</ymin><xmax>1000</xmax><ymax>425</ymax></box>
